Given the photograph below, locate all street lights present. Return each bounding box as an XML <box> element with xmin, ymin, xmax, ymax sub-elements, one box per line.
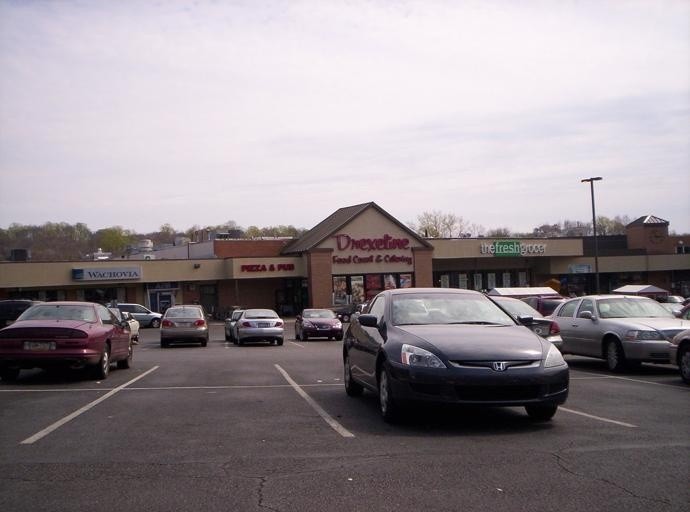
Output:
<box><xmin>581</xmin><ymin>177</ymin><xmax>603</xmax><ymax>294</ymax></box>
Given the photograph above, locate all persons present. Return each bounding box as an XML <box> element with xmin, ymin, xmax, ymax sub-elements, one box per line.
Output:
<box><xmin>678</xmin><ymin>296</ymin><xmax>690</xmax><ymax>321</ymax></box>
<box><xmin>562</xmin><ymin>289</ymin><xmax>578</xmax><ymax>300</ymax></box>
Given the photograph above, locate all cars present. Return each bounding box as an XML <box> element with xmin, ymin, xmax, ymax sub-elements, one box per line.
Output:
<box><xmin>343</xmin><ymin>288</ymin><xmax>569</xmax><ymax>421</ymax></box>
<box><xmin>0</xmin><ymin>300</ymin><xmax>141</xmax><ymax>380</ymax></box>
<box><xmin>106</xmin><ymin>302</ymin><xmax>163</xmax><ymax>328</ymax></box>
<box><xmin>295</xmin><ymin>304</ymin><xmax>355</xmax><ymax>341</ymax></box>
<box><xmin>159</xmin><ymin>305</ymin><xmax>212</xmax><ymax>347</ymax></box>
<box><xmin>543</xmin><ymin>294</ymin><xmax>690</xmax><ymax>385</ymax></box>
<box><xmin>224</xmin><ymin>308</ymin><xmax>287</xmax><ymax>346</ymax></box>
